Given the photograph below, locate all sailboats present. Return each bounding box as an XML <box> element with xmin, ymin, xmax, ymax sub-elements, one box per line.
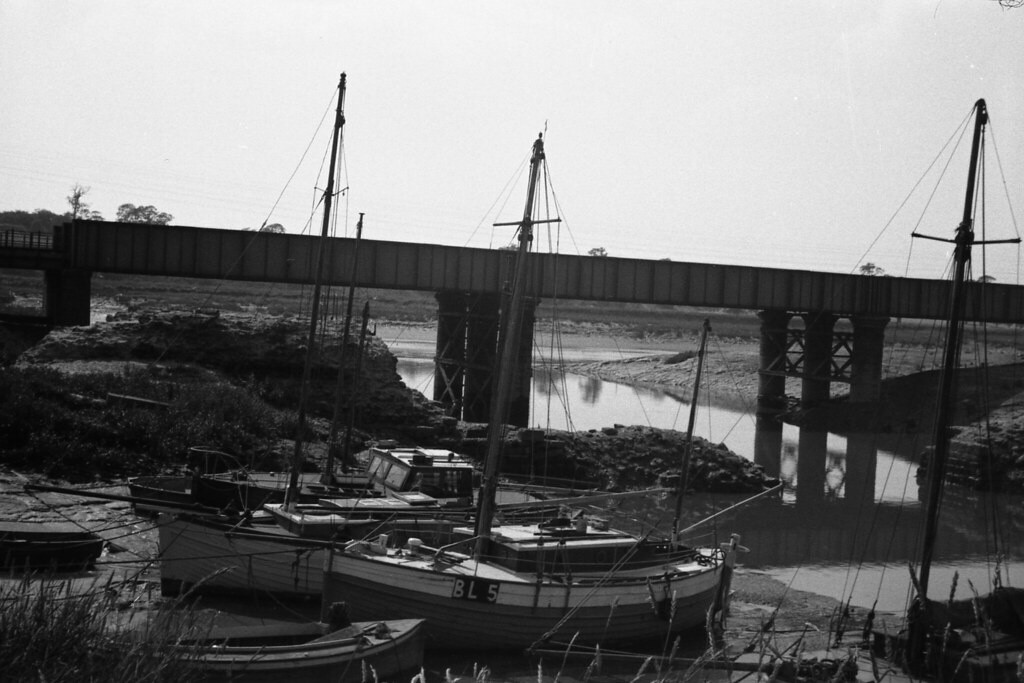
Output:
<box><xmin>0</xmin><ymin>73</ymin><xmax>1024</xmax><ymax>683</ymax></box>
